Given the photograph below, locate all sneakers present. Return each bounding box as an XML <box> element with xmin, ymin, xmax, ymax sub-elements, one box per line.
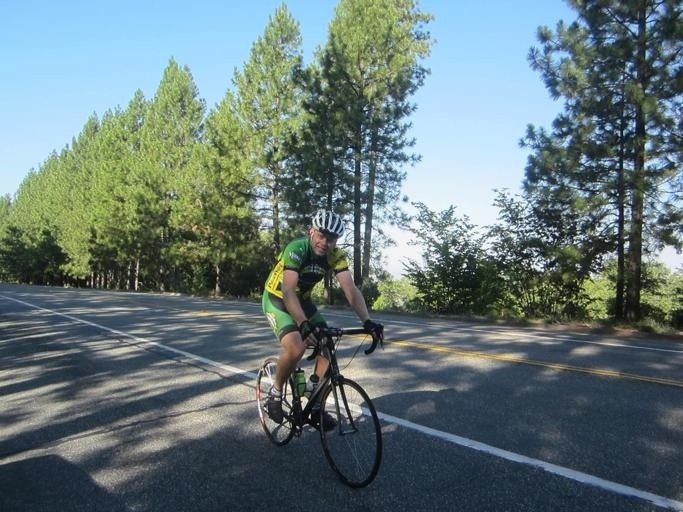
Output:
<box><xmin>268</xmin><ymin>387</ymin><xmax>283</xmax><ymax>424</ymax></box>
<box><xmin>311</xmin><ymin>412</ymin><xmax>338</xmax><ymax>428</ymax></box>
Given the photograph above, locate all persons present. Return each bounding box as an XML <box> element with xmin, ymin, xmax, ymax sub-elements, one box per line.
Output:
<box><xmin>259</xmin><ymin>207</ymin><xmax>383</xmax><ymax>434</ymax></box>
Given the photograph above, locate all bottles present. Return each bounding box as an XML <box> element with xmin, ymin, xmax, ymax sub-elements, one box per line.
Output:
<box><xmin>303</xmin><ymin>374</ymin><xmax>319</xmax><ymax>399</ymax></box>
<box><xmin>294</xmin><ymin>368</ymin><xmax>306</xmax><ymax>398</ymax></box>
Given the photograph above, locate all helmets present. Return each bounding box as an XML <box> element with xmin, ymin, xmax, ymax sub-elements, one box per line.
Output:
<box><xmin>312</xmin><ymin>209</ymin><xmax>345</xmax><ymax>238</ymax></box>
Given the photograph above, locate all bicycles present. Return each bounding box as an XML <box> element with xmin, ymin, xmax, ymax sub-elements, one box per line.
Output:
<box><xmin>255</xmin><ymin>322</ymin><xmax>383</xmax><ymax>489</ymax></box>
